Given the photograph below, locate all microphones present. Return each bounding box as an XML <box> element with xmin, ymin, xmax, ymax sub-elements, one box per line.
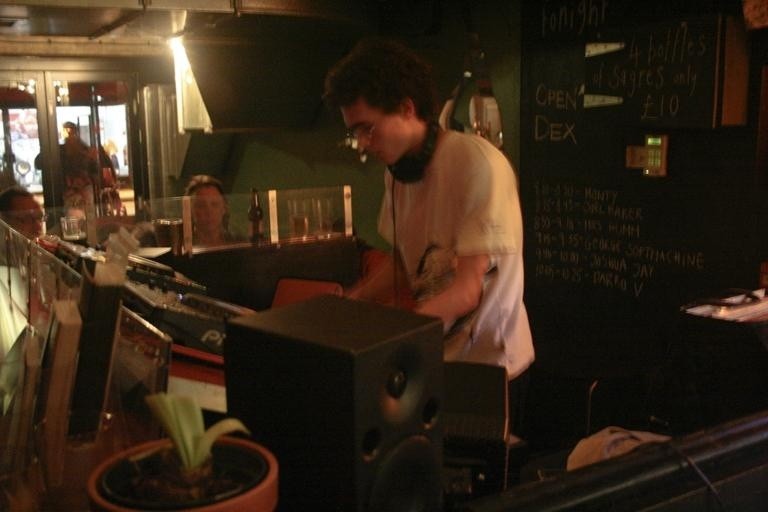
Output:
<box><xmin>648</xmin><ymin>414</ymin><xmax>669</xmax><ymax>429</ymax></box>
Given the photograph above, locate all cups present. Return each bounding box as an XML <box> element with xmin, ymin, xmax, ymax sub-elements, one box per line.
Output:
<box><xmin>286</xmin><ymin>197</ymin><xmax>334</xmax><ymax>240</ymax></box>
<box><xmin>59</xmin><ymin>216</ymin><xmax>87</xmax><ymax>248</ymax></box>
<box><xmin>151</xmin><ymin>219</ymin><xmax>184</xmax><ymax>258</ymax></box>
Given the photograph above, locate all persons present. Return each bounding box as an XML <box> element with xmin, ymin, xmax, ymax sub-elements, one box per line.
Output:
<box><xmin>165</xmin><ymin>174</ymin><xmax>242</xmax><ymax>249</ymax></box>
<box><xmin>2</xmin><ymin>188</ymin><xmax>49</xmax><ymax>480</ymax></box>
<box><xmin>36</xmin><ymin>121</ymin><xmax>131</xmax><ymax>238</ymax></box>
<box><xmin>321</xmin><ymin>41</ymin><xmax>538</xmax><ymax>483</ymax></box>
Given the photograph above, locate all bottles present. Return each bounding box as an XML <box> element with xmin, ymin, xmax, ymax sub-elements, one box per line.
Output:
<box><xmin>246</xmin><ymin>185</ymin><xmax>265</xmax><ymax>244</ymax></box>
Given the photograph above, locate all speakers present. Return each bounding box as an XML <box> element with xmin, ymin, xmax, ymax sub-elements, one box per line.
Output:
<box><xmin>224</xmin><ymin>294</ymin><xmax>447</xmax><ymax>510</ymax></box>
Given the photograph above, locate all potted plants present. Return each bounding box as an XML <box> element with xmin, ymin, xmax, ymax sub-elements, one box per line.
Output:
<box><xmin>86</xmin><ymin>392</ymin><xmax>280</xmax><ymax>512</ymax></box>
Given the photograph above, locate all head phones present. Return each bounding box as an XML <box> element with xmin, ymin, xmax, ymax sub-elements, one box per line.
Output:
<box><xmin>387</xmin><ymin>120</ymin><xmax>443</xmax><ymax>183</ymax></box>
